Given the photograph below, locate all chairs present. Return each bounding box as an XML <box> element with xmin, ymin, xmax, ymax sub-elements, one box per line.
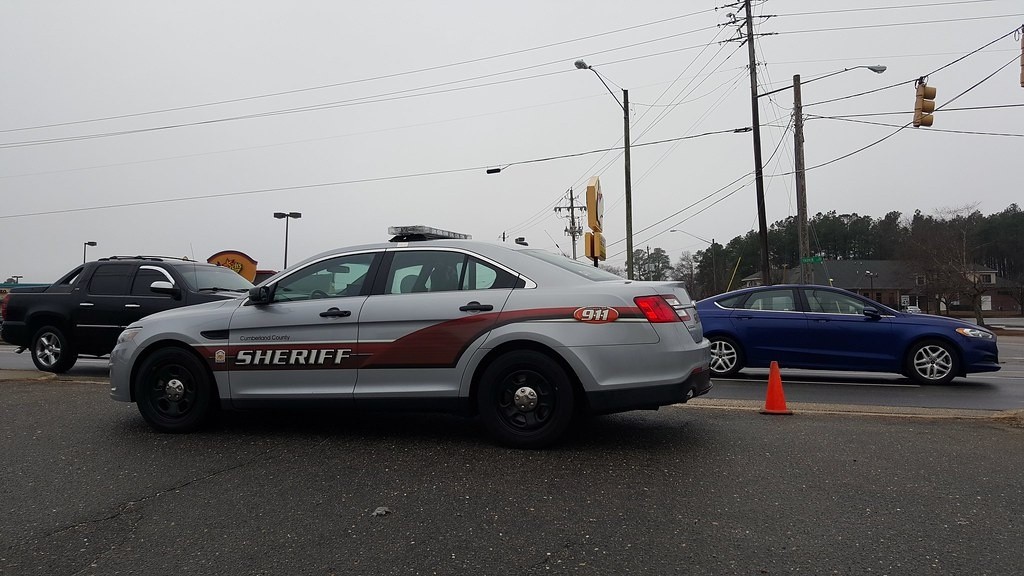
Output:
<box><xmin>400</xmin><ymin>275</ymin><xmax>426</xmax><ymax>292</ymax></box>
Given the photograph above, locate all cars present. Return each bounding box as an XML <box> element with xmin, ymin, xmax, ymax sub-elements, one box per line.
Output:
<box><xmin>0</xmin><ymin>256</ymin><xmax>310</xmax><ymax>380</ymax></box>
<box><xmin>109</xmin><ymin>226</ymin><xmax>715</xmax><ymax>453</ymax></box>
<box><xmin>900</xmin><ymin>306</ymin><xmax>922</xmax><ymax>314</ymax></box>
<box><xmin>694</xmin><ymin>282</ymin><xmax>1001</xmax><ymax>386</ymax></box>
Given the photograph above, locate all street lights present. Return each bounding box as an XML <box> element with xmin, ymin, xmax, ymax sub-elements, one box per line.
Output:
<box><xmin>750</xmin><ymin>65</ymin><xmax>886</xmax><ymax>308</ymax></box>
<box><xmin>12</xmin><ymin>276</ymin><xmax>23</xmax><ymax>284</ymax></box>
<box><xmin>83</xmin><ymin>241</ymin><xmax>97</xmax><ymax>263</ymax></box>
<box><xmin>671</xmin><ymin>230</ymin><xmax>715</xmax><ymax>279</ymax></box>
<box><xmin>864</xmin><ymin>269</ymin><xmax>879</xmax><ymax>300</ymax></box>
<box><xmin>575</xmin><ymin>58</ymin><xmax>633</xmax><ymax>281</ymax></box>
<box><xmin>274</xmin><ymin>211</ymin><xmax>301</xmax><ymax>271</ymax></box>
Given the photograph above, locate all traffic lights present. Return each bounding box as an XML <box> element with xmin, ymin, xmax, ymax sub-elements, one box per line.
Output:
<box><xmin>913</xmin><ymin>83</ymin><xmax>936</xmax><ymax>127</ymax></box>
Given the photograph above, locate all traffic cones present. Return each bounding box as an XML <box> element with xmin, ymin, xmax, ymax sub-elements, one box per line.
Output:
<box><xmin>760</xmin><ymin>361</ymin><xmax>795</xmax><ymax>415</ymax></box>
